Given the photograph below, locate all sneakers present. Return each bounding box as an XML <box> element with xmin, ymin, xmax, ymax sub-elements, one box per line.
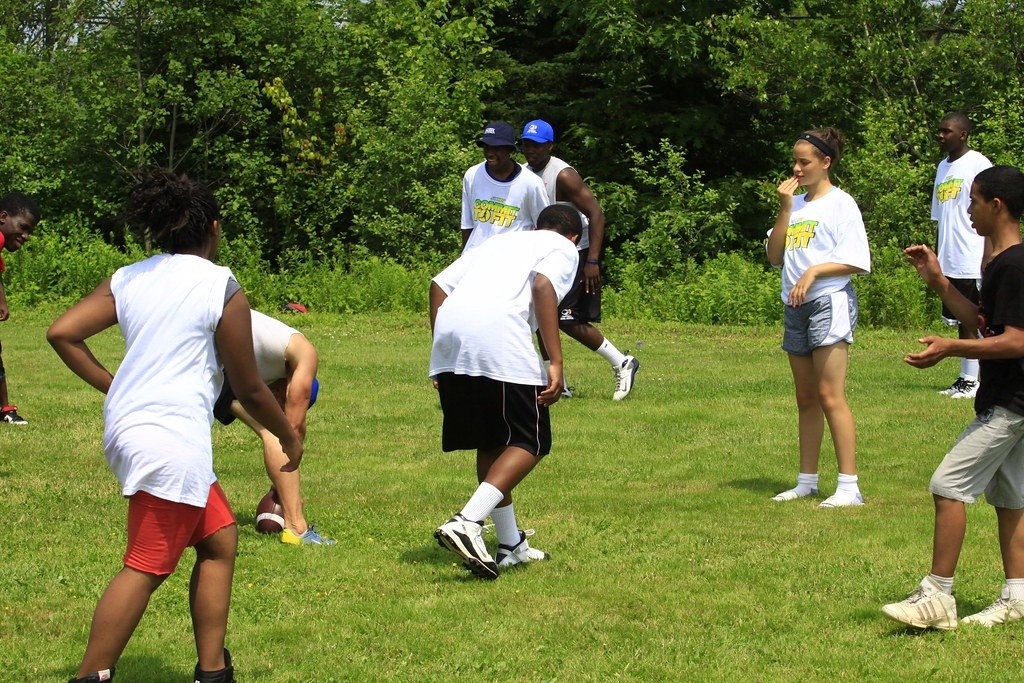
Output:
<box><xmin>67</xmin><ymin>667</ymin><xmax>116</xmax><ymax>683</ymax></box>
<box><xmin>950</xmin><ymin>374</ymin><xmax>980</xmax><ymax>399</ymax></box>
<box><xmin>281</xmin><ymin>526</ymin><xmax>338</xmax><ymax>548</ymax></box>
<box><xmin>495</xmin><ymin>529</ymin><xmax>551</xmax><ymax>572</ymax></box>
<box><xmin>611</xmin><ymin>350</ymin><xmax>639</xmax><ymax>403</ymax></box>
<box><xmin>561</xmin><ymin>383</ymin><xmax>576</xmax><ymax>398</ymax></box>
<box><xmin>959</xmin><ymin>584</ymin><xmax>1024</xmax><ymax>630</ymax></box>
<box><xmin>0</xmin><ymin>405</ymin><xmax>28</xmax><ymax>426</ymax></box>
<box><xmin>882</xmin><ymin>575</ymin><xmax>958</xmax><ymax>632</ymax></box>
<box><xmin>433</xmin><ymin>512</ymin><xmax>500</xmax><ymax>582</ymax></box>
<box><xmin>193</xmin><ymin>647</ymin><xmax>237</xmax><ymax>683</ymax></box>
<box><xmin>937</xmin><ymin>372</ymin><xmax>967</xmax><ymax>396</ymax></box>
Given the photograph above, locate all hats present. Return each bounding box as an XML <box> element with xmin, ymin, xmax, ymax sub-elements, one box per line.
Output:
<box><xmin>518</xmin><ymin>119</ymin><xmax>554</xmax><ymax>143</ymax></box>
<box><xmin>476</xmin><ymin>121</ymin><xmax>519</xmax><ymax>153</ymax></box>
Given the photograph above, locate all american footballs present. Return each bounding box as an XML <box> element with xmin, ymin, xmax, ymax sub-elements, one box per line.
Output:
<box><xmin>255</xmin><ymin>488</ymin><xmax>305</xmax><ymax>535</ymax></box>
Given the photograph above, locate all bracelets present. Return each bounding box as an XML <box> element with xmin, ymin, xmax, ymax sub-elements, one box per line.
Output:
<box><xmin>586</xmin><ymin>259</ymin><xmax>598</xmax><ymax>264</ymax></box>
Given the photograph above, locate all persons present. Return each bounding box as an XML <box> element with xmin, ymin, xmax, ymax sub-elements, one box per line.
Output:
<box><xmin>0</xmin><ymin>193</ymin><xmax>41</xmax><ymax>424</ymax></box>
<box><xmin>47</xmin><ymin>168</ymin><xmax>304</xmax><ymax>683</ymax></box>
<box><xmin>880</xmin><ymin>167</ymin><xmax>1024</xmax><ymax>631</ymax></box>
<box><xmin>428</xmin><ymin>204</ymin><xmax>583</xmax><ymax>585</ymax></box>
<box><xmin>931</xmin><ymin>113</ymin><xmax>993</xmax><ymax>396</ymax></box>
<box><xmin>213</xmin><ymin>307</ymin><xmax>331</xmax><ymax>543</ymax></box>
<box><xmin>461</xmin><ymin>122</ymin><xmax>551</xmax><ymax>250</ymax></box>
<box><xmin>766</xmin><ymin>127</ymin><xmax>870</xmax><ymax>507</ymax></box>
<box><xmin>520</xmin><ymin>120</ymin><xmax>639</xmax><ymax>402</ymax></box>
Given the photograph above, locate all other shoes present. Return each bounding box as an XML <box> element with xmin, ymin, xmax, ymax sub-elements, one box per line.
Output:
<box><xmin>772</xmin><ymin>485</ymin><xmax>821</xmax><ymax>503</ymax></box>
<box><xmin>816</xmin><ymin>490</ymin><xmax>865</xmax><ymax>511</ymax></box>
